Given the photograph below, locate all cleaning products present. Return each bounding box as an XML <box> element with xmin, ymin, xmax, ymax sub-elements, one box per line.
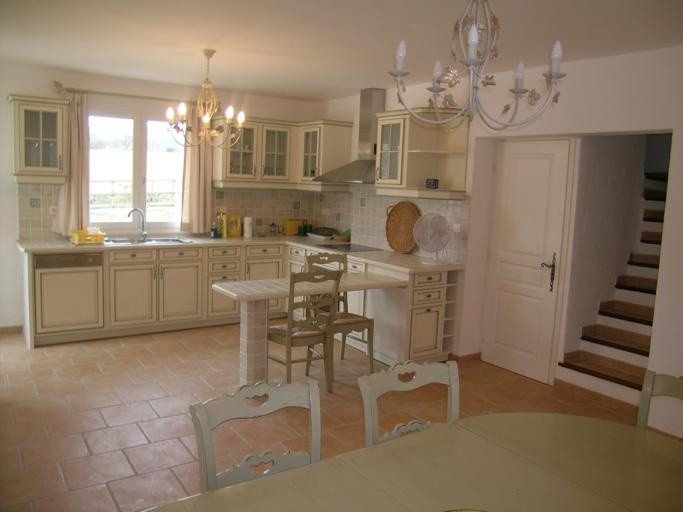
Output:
<box><xmin>219</xmin><ymin>212</ymin><xmax>227</xmax><ymax>237</ymax></box>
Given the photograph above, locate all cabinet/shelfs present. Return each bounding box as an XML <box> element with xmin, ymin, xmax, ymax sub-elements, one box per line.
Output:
<box><xmin>338</xmin><ymin>256</ymin><xmax>365</xmax><ymax>343</ymax></box>
<box><xmin>377</xmin><ymin>108</ymin><xmax>471</xmax><ymax>200</ymax></box>
<box><xmin>108</xmin><ymin>250</ymin><xmax>203</xmax><ymax>336</ymax></box>
<box><xmin>208</xmin><ymin>246</ymin><xmax>244</xmax><ymax>326</ymax></box>
<box><xmin>286</xmin><ymin>245</ymin><xmax>306</xmax><ymax>318</ymax></box>
<box><xmin>245</xmin><ymin>246</ymin><xmax>284</xmax><ymax>320</ymax></box>
<box><xmin>10</xmin><ymin>93</ymin><xmax>70</xmax><ymax>184</ymax></box>
<box><xmin>408</xmin><ymin>275</ymin><xmax>460</xmax><ymax>363</ymax></box>
<box><xmin>299</xmin><ymin>121</ymin><xmax>353</xmax><ymax>196</ymax></box>
<box><xmin>24</xmin><ymin>253</ymin><xmax>106</xmax><ymax>349</ymax></box>
<box><xmin>212</xmin><ymin>116</ymin><xmax>296</xmax><ymax>191</ymax></box>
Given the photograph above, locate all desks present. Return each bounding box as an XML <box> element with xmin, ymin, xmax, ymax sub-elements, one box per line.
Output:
<box><xmin>149</xmin><ymin>413</ymin><xmax>681</xmax><ymax>512</ymax></box>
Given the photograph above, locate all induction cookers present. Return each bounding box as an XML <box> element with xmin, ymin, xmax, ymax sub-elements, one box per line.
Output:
<box><xmin>315</xmin><ymin>243</ymin><xmax>385</xmax><ymax>254</ymax></box>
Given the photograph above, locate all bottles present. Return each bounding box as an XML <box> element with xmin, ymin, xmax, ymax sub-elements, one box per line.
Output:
<box><xmin>208</xmin><ymin>209</ymin><xmax>227</xmax><ymax>239</ymax></box>
<box><xmin>46</xmin><ymin>142</ymin><xmax>57</xmax><ymax>168</ymax></box>
<box><xmin>29</xmin><ymin>142</ymin><xmax>40</xmax><ymax>166</ymax></box>
<box><xmin>269</xmin><ymin>222</ymin><xmax>276</xmax><ymax>233</ymax></box>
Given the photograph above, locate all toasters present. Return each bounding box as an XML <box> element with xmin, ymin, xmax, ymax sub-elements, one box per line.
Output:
<box><xmin>282</xmin><ymin>218</ymin><xmax>306</xmax><ymax>235</ymax></box>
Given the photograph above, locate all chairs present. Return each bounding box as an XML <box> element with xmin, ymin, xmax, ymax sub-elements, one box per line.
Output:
<box><xmin>639</xmin><ymin>357</ymin><xmax>682</xmax><ymax>445</ymax></box>
<box><xmin>358</xmin><ymin>361</ymin><xmax>459</xmax><ymax>444</ymax></box>
<box><xmin>269</xmin><ymin>271</ymin><xmax>336</xmax><ymax>393</ymax></box>
<box><xmin>190</xmin><ymin>383</ymin><xmax>321</xmax><ymax>497</ymax></box>
<box><xmin>306</xmin><ymin>251</ymin><xmax>375</xmax><ymax>382</ymax></box>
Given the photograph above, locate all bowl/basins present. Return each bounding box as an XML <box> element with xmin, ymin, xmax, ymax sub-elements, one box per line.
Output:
<box><xmin>333</xmin><ymin>235</ymin><xmax>350</xmax><ymax>243</ymax></box>
<box><xmin>306</xmin><ymin>233</ymin><xmax>332</xmax><ymax>242</ymax></box>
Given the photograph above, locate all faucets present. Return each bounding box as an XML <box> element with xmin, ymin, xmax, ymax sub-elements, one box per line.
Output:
<box><xmin>127</xmin><ymin>208</ymin><xmax>148</xmax><ymax>242</ymax></box>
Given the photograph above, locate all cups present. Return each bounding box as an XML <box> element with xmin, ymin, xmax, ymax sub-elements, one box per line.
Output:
<box><xmin>228</xmin><ymin>141</ymin><xmax>252</xmax><ymax>175</ymax></box>
<box><xmin>298</xmin><ymin>225</ymin><xmax>306</xmax><ymax>236</ymax></box>
<box><xmin>306</xmin><ymin>224</ymin><xmax>312</xmax><ymax>234</ymax></box>
<box><xmin>242</xmin><ymin>217</ymin><xmax>253</xmax><ymax>239</ymax></box>
<box><xmin>425</xmin><ymin>178</ymin><xmax>439</xmax><ymax>188</ymax></box>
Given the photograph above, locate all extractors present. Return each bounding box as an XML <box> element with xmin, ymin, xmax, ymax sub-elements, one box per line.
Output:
<box><xmin>312</xmin><ymin>87</ymin><xmax>386</xmax><ymax>184</ymax></box>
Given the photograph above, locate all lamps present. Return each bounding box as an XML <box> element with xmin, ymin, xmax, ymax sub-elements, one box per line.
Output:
<box><xmin>165</xmin><ymin>50</ymin><xmax>242</xmax><ymax>149</ymax></box>
<box><xmin>390</xmin><ymin>1</ymin><xmax>567</xmax><ymax>130</ymax></box>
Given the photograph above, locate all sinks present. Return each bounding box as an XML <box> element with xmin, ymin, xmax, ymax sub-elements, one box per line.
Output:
<box><xmin>145</xmin><ymin>238</ymin><xmax>193</xmax><ymax>244</ymax></box>
<box><xmin>103</xmin><ymin>238</ymin><xmax>148</xmax><ymax>245</ymax></box>
